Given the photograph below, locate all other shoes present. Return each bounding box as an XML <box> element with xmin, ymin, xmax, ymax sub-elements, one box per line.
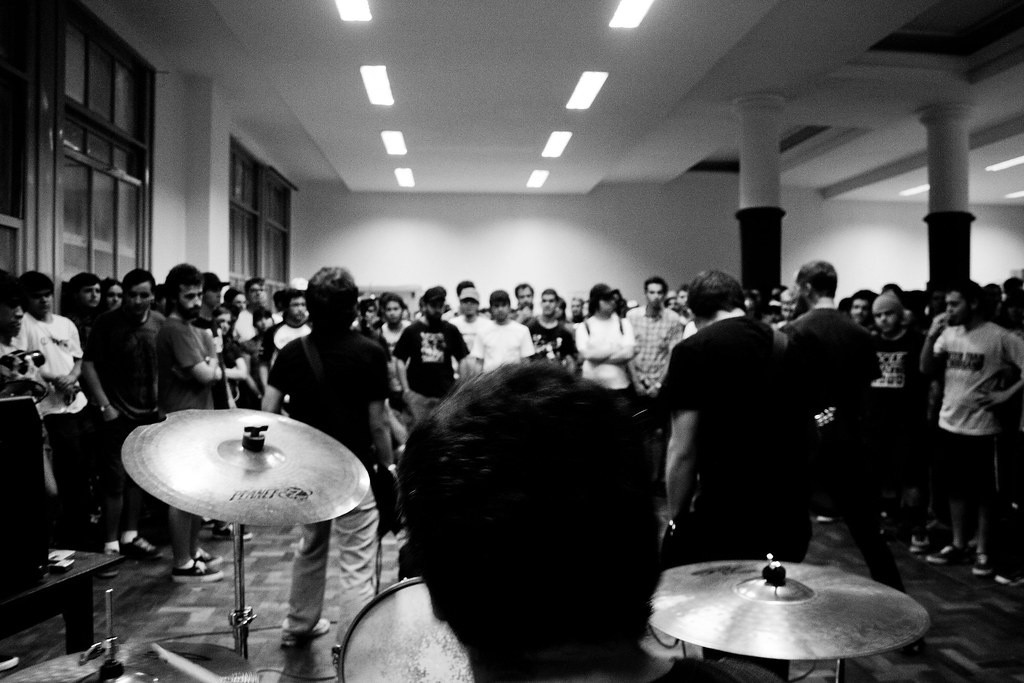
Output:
<box><xmin>0</xmin><ymin>654</ymin><xmax>19</xmax><ymax>671</ymax></box>
<box><xmin>994</xmin><ymin>573</ymin><xmax>1024</xmax><ymax>587</ymax></box>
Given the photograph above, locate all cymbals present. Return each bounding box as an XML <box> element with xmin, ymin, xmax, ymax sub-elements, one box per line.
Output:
<box><xmin>1</xmin><ymin>640</ymin><xmax>259</xmax><ymax>683</ymax></box>
<box><xmin>121</xmin><ymin>406</ymin><xmax>371</xmax><ymax>527</ymax></box>
<box><xmin>646</xmin><ymin>559</ymin><xmax>930</xmax><ymax>659</ymax></box>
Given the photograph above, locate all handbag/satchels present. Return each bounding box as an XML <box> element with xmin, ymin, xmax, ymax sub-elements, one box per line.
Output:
<box><xmin>370</xmin><ymin>462</ymin><xmax>407</xmax><ymax>541</ymax></box>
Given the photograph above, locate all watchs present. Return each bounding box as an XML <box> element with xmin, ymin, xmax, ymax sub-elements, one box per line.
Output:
<box><xmin>99</xmin><ymin>403</ymin><xmax>110</xmax><ymax>411</ymax></box>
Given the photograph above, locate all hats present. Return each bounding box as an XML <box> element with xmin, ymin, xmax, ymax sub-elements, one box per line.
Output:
<box><xmin>590</xmin><ymin>284</ymin><xmax>620</xmax><ymax>299</ymax></box>
<box><xmin>459</xmin><ymin>287</ymin><xmax>480</xmax><ymax>305</ymax></box>
<box><xmin>490</xmin><ymin>290</ymin><xmax>510</xmax><ymax>304</ymax></box>
<box><xmin>422</xmin><ymin>286</ymin><xmax>446</xmax><ymax>302</ymax></box>
<box><xmin>201</xmin><ymin>272</ymin><xmax>230</xmax><ymax>290</ymax></box>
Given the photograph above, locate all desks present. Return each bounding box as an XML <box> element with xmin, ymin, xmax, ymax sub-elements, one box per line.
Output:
<box><xmin>0</xmin><ymin>550</ymin><xmax>124</xmax><ymax>654</ymax></box>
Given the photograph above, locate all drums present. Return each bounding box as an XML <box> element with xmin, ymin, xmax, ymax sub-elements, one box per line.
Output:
<box><xmin>337</xmin><ymin>575</ymin><xmax>471</xmax><ymax>682</ymax></box>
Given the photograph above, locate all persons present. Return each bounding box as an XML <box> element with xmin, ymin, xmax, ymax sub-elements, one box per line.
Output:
<box><xmin>0</xmin><ymin>269</ymin><xmax>54</xmax><ymax>676</ymax></box>
<box><xmin>659</xmin><ymin>268</ymin><xmax>811</xmax><ymax>682</ymax></box>
<box><xmin>18</xmin><ymin>260</ymin><xmax>1024</xmax><ymax>586</ymax></box>
<box><xmin>260</xmin><ymin>264</ymin><xmax>394</xmax><ymax>662</ymax></box>
<box><xmin>397</xmin><ymin>355</ymin><xmax>789</xmax><ymax>683</ymax></box>
<box><xmin>775</xmin><ymin>260</ymin><xmax>932</xmax><ymax>658</ymax></box>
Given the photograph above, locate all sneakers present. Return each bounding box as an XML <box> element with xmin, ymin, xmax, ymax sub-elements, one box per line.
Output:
<box><xmin>280</xmin><ymin>618</ymin><xmax>330</xmax><ymax>647</ymax></box>
<box><xmin>120</xmin><ymin>537</ymin><xmax>164</xmax><ymax>561</ymax></box>
<box><xmin>972</xmin><ymin>554</ymin><xmax>994</xmax><ymax>576</ymax></box>
<box><xmin>96</xmin><ymin>550</ymin><xmax>121</xmax><ymax>577</ymax></box>
<box><xmin>925</xmin><ymin>545</ymin><xmax>971</xmax><ymax>565</ymax></box>
<box><xmin>193</xmin><ymin>548</ymin><xmax>224</xmax><ymax>566</ymax></box>
<box><xmin>171</xmin><ymin>560</ymin><xmax>224</xmax><ymax>584</ymax></box>
